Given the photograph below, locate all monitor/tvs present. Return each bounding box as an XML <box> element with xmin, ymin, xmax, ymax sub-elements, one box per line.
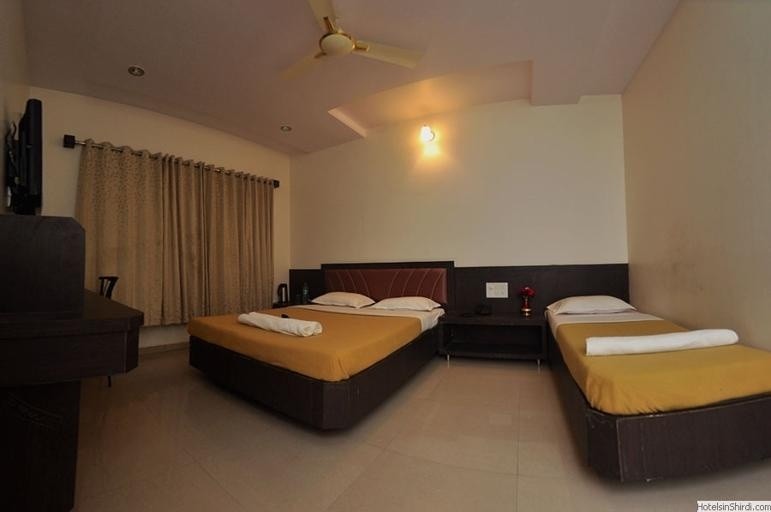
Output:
<box><xmin>6</xmin><ymin>98</ymin><xmax>43</xmax><ymax>216</ymax></box>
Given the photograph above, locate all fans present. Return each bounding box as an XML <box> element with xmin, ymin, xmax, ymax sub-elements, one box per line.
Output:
<box><xmin>263</xmin><ymin>0</ymin><xmax>422</xmax><ymax>87</ymax></box>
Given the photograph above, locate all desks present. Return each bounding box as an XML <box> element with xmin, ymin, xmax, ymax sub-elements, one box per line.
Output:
<box><xmin>0</xmin><ymin>288</ymin><xmax>146</xmax><ymax>512</ymax></box>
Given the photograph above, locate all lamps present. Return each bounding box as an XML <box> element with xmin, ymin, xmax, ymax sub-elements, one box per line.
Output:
<box><xmin>516</xmin><ymin>285</ymin><xmax>536</xmax><ymax>321</ymax></box>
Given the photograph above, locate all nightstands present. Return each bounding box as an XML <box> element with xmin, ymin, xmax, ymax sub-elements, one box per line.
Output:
<box><xmin>437</xmin><ymin>310</ymin><xmax>548</xmax><ymax>366</ymax></box>
<box><xmin>271</xmin><ymin>301</ymin><xmax>311</xmax><ymax>309</ymax></box>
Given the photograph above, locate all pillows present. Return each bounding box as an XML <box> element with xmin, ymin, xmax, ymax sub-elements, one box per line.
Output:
<box><xmin>545</xmin><ymin>294</ymin><xmax>638</xmax><ymax>316</ymax></box>
<box><xmin>310</xmin><ymin>292</ymin><xmax>375</xmax><ymax>308</ymax></box>
<box><xmin>368</xmin><ymin>296</ymin><xmax>441</xmax><ymax>311</ymax></box>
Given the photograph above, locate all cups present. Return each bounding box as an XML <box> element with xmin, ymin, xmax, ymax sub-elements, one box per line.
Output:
<box><xmin>278</xmin><ymin>283</ymin><xmax>289</xmax><ymax>304</ymax></box>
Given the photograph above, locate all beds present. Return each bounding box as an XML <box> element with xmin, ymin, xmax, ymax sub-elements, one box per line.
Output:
<box><xmin>186</xmin><ymin>261</ymin><xmax>456</xmax><ymax>432</ymax></box>
<box><xmin>544</xmin><ymin>307</ymin><xmax>771</xmax><ymax>486</ymax></box>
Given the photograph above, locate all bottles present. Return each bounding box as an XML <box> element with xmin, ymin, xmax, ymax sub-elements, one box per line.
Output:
<box><xmin>303</xmin><ymin>282</ymin><xmax>309</xmax><ymax>303</ymax></box>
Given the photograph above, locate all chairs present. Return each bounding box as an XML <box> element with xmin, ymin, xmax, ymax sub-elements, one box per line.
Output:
<box><xmin>96</xmin><ymin>276</ymin><xmax>119</xmax><ymax>389</ymax></box>
